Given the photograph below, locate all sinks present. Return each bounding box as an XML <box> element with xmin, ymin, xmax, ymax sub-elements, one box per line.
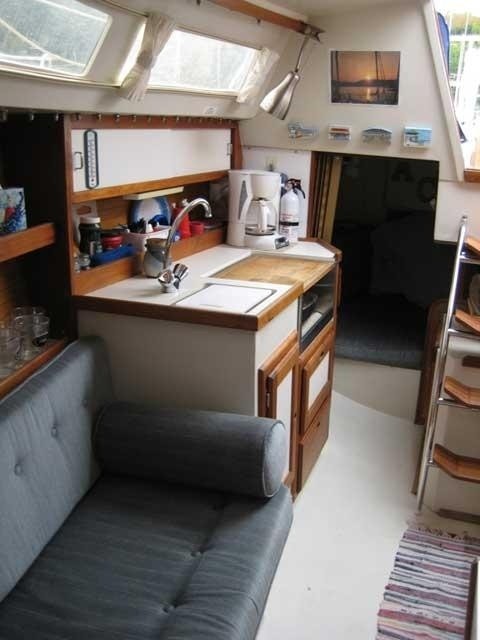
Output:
<box><xmin>170</xmin><ymin>281</ymin><xmax>277</xmax><ymax>315</ymax></box>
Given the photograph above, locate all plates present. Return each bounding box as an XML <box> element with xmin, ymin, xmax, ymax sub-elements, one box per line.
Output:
<box><xmin>130</xmin><ymin>195</ymin><xmax>172</xmax><ymax>234</ymax></box>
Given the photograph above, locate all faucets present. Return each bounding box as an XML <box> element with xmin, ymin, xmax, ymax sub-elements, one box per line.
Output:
<box><xmin>156</xmin><ymin>197</ymin><xmax>214</xmax><ymax>292</ymax></box>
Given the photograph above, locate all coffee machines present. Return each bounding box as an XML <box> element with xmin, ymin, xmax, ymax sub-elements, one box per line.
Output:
<box><xmin>226</xmin><ymin>168</ymin><xmax>290</xmax><ymax>251</ymax></box>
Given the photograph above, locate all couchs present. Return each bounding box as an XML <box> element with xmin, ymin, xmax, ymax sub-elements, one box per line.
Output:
<box><xmin>0</xmin><ymin>335</ymin><xmax>293</xmax><ymax>640</ymax></box>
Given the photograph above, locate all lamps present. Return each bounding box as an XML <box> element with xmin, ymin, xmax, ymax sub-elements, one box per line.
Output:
<box><xmin>258</xmin><ymin>25</ymin><xmax>325</xmax><ymax>121</ymax></box>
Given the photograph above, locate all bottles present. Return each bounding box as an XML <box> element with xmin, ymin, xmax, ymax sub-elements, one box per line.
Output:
<box><xmin>175</xmin><ymin>206</ymin><xmax>191</xmax><ymax>238</ymax></box>
<box><xmin>74</xmin><ymin>214</ymin><xmax>125</xmax><ymax>275</ymax></box>
<box><xmin>142</xmin><ymin>237</ymin><xmax>169</xmax><ymax>278</ymax></box>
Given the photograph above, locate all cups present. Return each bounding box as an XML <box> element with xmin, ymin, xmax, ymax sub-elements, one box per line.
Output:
<box><xmin>191</xmin><ymin>221</ymin><xmax>204</xmax><ymax>236</ymax></box>
<box><xmin>1</xmin><ymin>188</ymin><xmax>28</xmax><ymax>237</ymax></box>
<box><xmin>1</xmin><ymin>301</ymin><xmax>51</xmax><ymax>382</ymax></box>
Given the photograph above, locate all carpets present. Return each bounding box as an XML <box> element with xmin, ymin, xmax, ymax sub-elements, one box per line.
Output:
<box><xmin>376</xmin><ymin>521</ymin><xmax>480</xmax><ymax>640</ymax></box>
